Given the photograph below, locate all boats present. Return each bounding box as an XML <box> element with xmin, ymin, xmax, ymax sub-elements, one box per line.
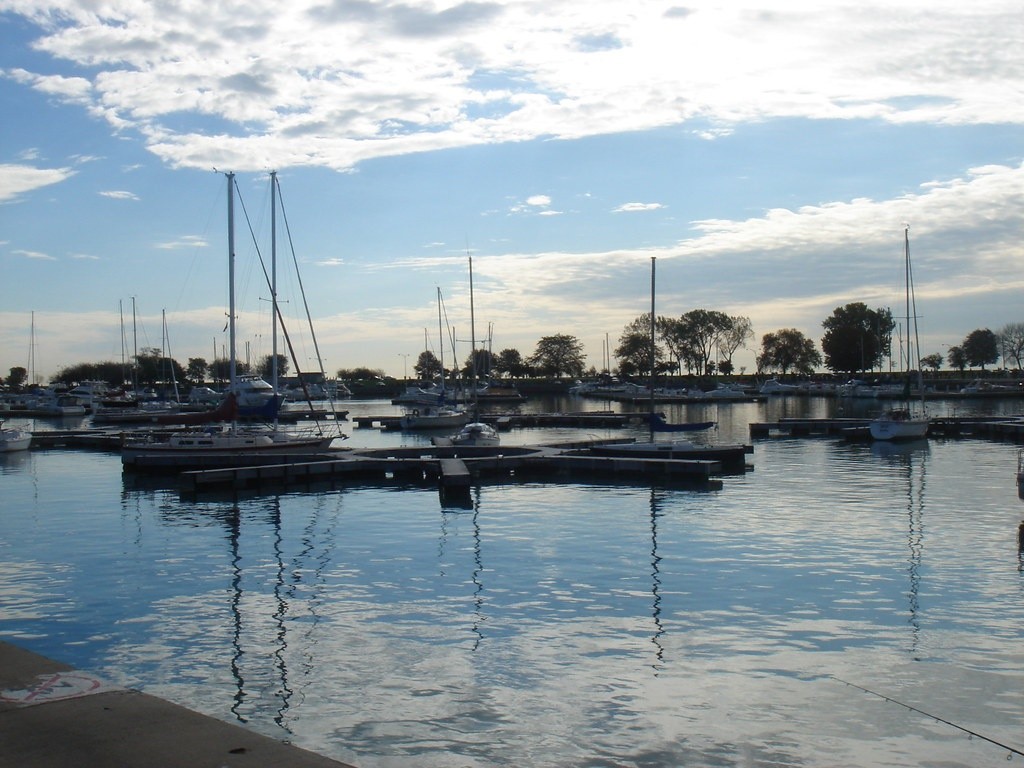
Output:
<box><xmin>187</xmin><ymin>386</ymin><xmax>223</xmax><ymax>403</ymax></box>
<box><xmin>68</xmin><ymin>379</ymin><xmax>110</xmax><ymax>401</ymax></box>
<box><xmin>393</xmin><ymin>337</ymin><xmax>444</xmax><ymax>406</ymax></box>
<box><xmin>48</xmin><ymin>392</ymin><xmax>87</xmax><ymax>417</ymax></box>
<box><xmin>962</xmin><ymin>378</ymin><xmax>1024</xmax><ymax>400</ymax></box>
<box><xmin>706</xmin><ymin>380</ymin><xmax>758</xmax><ymax>399</ymax></box>
<box><xmin>221</xmin><ymin>342</ymin><xmax>287</xmax><ymax>417</ymax></box>
<box><xmin>0</xmin><ymin>419</ymin><xmax>34</xmax><ymax>452</ymax></box>
<box><xmin>759</xmin><ymin>379</ymin><xmax>840</xmax><ymax>399</ymax></box>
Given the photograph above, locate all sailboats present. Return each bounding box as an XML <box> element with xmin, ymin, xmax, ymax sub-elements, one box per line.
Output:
<box><xmin>450</xmin><ymin>257</ymin><xmax>500</xmax><ymax>447</ymax></box>
<box><xmin>840</xmin><ymin>318</ymin><xmax>918</xmax><ymax>397</ymax></box>
<box><xmin>120</xmin><ymin>167</ymin><xmax>351</xmax><ymax>467</ymax></box>
<box><xmin>870</xmin><ymin>227</ymin><xmax>928</xmax><ymax>441</ymax></box>
<box><xmin>472</xmin><ymin>326</ymin><xmax>525</xmax><ymax>401</ymax></box>
<box><xmin>401</xmin><ymin>327</ymin><xmax>470</xmax><ymax>429</ymax></box>
<box><xmin>8</xmin><ymin>310</ymin><xmax>52</xmax><ymax>406</ymax></box>
<box><xmin>94</xmin><ymin>296</ymin><xmax>187</xmax><ymax>412</ymax></box>
<box><xmin>596</xmin><ymin>333</ymin><xmax>626</xmax><ymax>392</ymax></box>
